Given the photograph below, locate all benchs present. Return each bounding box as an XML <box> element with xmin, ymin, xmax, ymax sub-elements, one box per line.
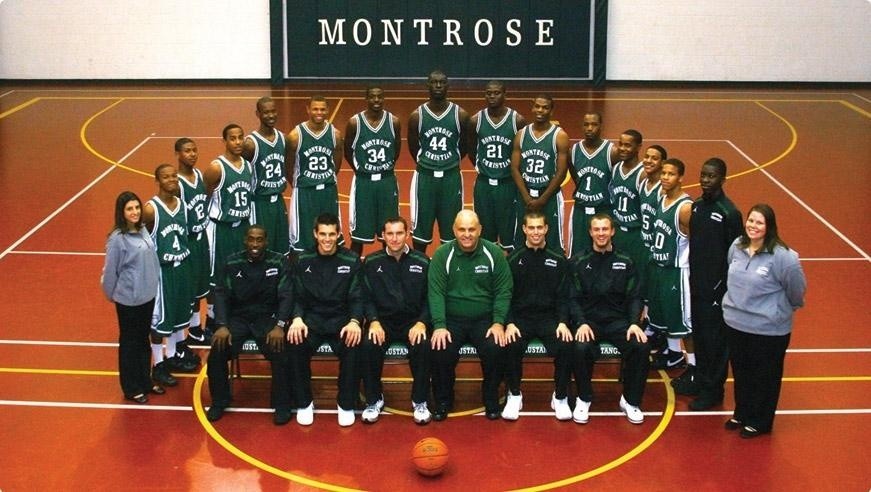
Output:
<box><xmin>222</xmin><ymin>338</ymin><xmax>659</xmax><ymax>399</ymax></box>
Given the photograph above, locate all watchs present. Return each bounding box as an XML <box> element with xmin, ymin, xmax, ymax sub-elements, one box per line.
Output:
<box><xmin>275</xmin><ymin>320</ymin><xmax>288</xmax><ymax>333</ymax></box>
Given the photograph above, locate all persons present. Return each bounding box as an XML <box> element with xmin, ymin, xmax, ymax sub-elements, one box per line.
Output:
<box><xmin>501</xmin><ymin>212</ymin><xmax>573</xmax><ymax>421</ymax></box>
<box><xmin>565</xmin><ymin>212</ymin><xmax>652</xmax><ymax>424</ymax></box>
<box><xmin>203</xmin><ymin>122</ymin><xmax>256</xmax><ymax>287</ymax></box>
<box><xmin>566</xmin><ymin>110</ymin><xmax>620</xmax><ymax>259</ymax></box>
<box><xmin>649</xmin><ymin>157</ymin><xmax>694</xmax><ymax>370</ymax></box>
<box><xmin>142</xmin><ymin>162</ymin><xmax>200</xmax><ymax>387</ymax></box>
<box><xmin>100</xmin><ymin>191</ymin><xmax>167</xmax><ymax>403</ymax></box>
<box><xmin>240</xmin><ymin>96</ymin><xmax>290</xmax><ymax>259</ymax></box>
<box><xmin>686</xmin><ymin>158</ymin><xmax>746</xmax><ymax>410</ymax></box>
<box><xmin>206</xmin><ymin>225</ymin><xmax>295</xmax><ymax>425</ymax></box>
<box><xmin>286</xmin><ymin>93</ymin><xmax>344</xmax><ymax>255</ymax></box>
<box><xmin>427</xmin><ymin>209</ymin><xmax>512</xmax><ymax>421</ymax></box>
<box><xmin>286</xmin><ymin>211</ymin><xmax>365</xmax><ymax>427</ymax></box>
<box><xmin>173</xmin><ymin>137</ymin><xmax>214</xmax><ymax>363</ymax></box>
<box><xmin>343</xmin><ymin>68</ymin><xmax>527</xmax><ymax>258</ymax></box>
<box><xmin>359</xmin><ymin>216</ymin><xmax>432</xmax><ymax>424</ymax></box>
<box><xmin>509</xmin><ymin>92</ymin><xmax>572</xmax><ymax>258</ymax></box>
<box><xmin>608</xmin><ymin>128</ymin><xmax>649</xmax><ymax>326</ymax></box>
<box><xmin>634</xmin><ymin>144</ymin><xmax>667</xmax><ymax>332</ymax></box>
<box><xmin>722</xmin><ymin>203</ymin><xmax>807</xmax><ymax>438</ymax></box>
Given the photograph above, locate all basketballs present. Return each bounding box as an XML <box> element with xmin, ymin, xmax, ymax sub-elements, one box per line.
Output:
<box><xmin>412</xmin><ymin>437</ymin><xmax>449</xmax><ymax>477</ymax></box>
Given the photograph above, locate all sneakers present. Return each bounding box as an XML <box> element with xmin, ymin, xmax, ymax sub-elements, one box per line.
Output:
<box><xmin>500</xmin><ymin>388</ymin><xmax>524</xmax><ymax>421</ymax></box>
<box><xmin>619</xmin><ymin>395</ymin><xmax>645</xmax><ymax>425</ymax></box>
<box><xmin>550</xmin><ymin>390</ymin><xmax>573</xmax><ymax>421</ymax></box>
<box><xmin>432</xmin><ymin>400</ymin><xmax>449</xmax><ymax>421</ymax></box>
<box><xmin>411</xmin><ymin>399</ymin><xmax>432</xmax><ymax>424</ymax></box>
<box><xmin>132</xmin><ymin>314</ymin><xmax>212</xmax><ymax>403</ymax></box>
<box><xmin>650</xmin><ymin>349</ymin><xmax>761</xmax><ymax>439</ymax></box>
<box><xmin>572</xmin><ymin>395</ymin><xmax>592</xmax><ymax>424</ymax></box>
<box><xmin>295</xmin><ymin>401</ymin><xmax>315</xmax><ymax>425</ymax></box>
<box><xmin>206</xmin><ymin>399</ymin><xmax>231</xmax><ymax>421</ymax></box>
<box><xmin>361</xmin><ymin>393</ymin><xmax>385</xmax><ymax>423</ymax></box>
<box><xmin>336</xmin><ymin>403</ymin><xmax>356</xmax><ymax>427</ymax></box>
<box><xmin>485</xmin><ymin>398</ymin><xmax>500</xmax><ymax>419</ymax></box>
<box><xmin>272</xmin><ymin>404</ymin><xmax>291</xmax><ymax>424</ymax></box>
